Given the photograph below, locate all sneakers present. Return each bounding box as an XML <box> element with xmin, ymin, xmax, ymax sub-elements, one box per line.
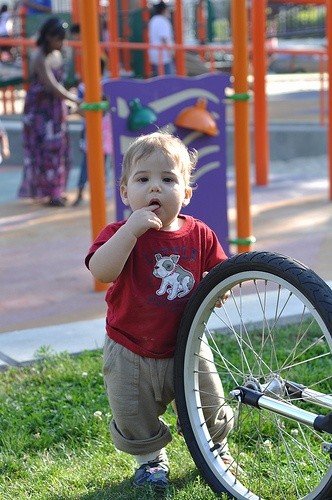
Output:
<box><xmin>220</xmin><ymin>452</ymin><xmax>244</xmax><ymax>479</ymax></box>
<box><xmin>130</xmin><ymin>460</ymin><xmax>172</xmax><ymax>491</ymax></box>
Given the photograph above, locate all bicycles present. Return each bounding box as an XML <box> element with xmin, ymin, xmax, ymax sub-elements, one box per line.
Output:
<box><xmin>171</xmin><ymin>250</ymin><xmax>332</xmax><ymax>500</ymax></box>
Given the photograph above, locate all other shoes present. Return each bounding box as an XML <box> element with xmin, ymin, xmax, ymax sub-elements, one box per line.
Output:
<box><xmin>49</xmin><ymin>196</ymin><xmax>69</xmax><ymax>205</ymax></box>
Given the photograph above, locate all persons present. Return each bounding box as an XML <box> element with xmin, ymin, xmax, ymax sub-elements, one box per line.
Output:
<box><xmin>16</xmin><ymin>17</ymin><xmax>82</xmax><ymax>206</ymax></box>
<box><xmin>84</xmin><ymin>131</ymin><xmax>246</xmax><ymax>496</ymax></box>
<box><xmin>143</xmin><ymin>1</ymin><xmax>175</xmax><ymax>77</ymax></box>
<box><xmin>0</xmin><ymin>4</ymin><xmax>16</xmax><ymax>64</ymax></box>
<box><xmin>98</xmin><ymin>0</ymin><xmax>112</xmax><ymax>78</ymax></box>
